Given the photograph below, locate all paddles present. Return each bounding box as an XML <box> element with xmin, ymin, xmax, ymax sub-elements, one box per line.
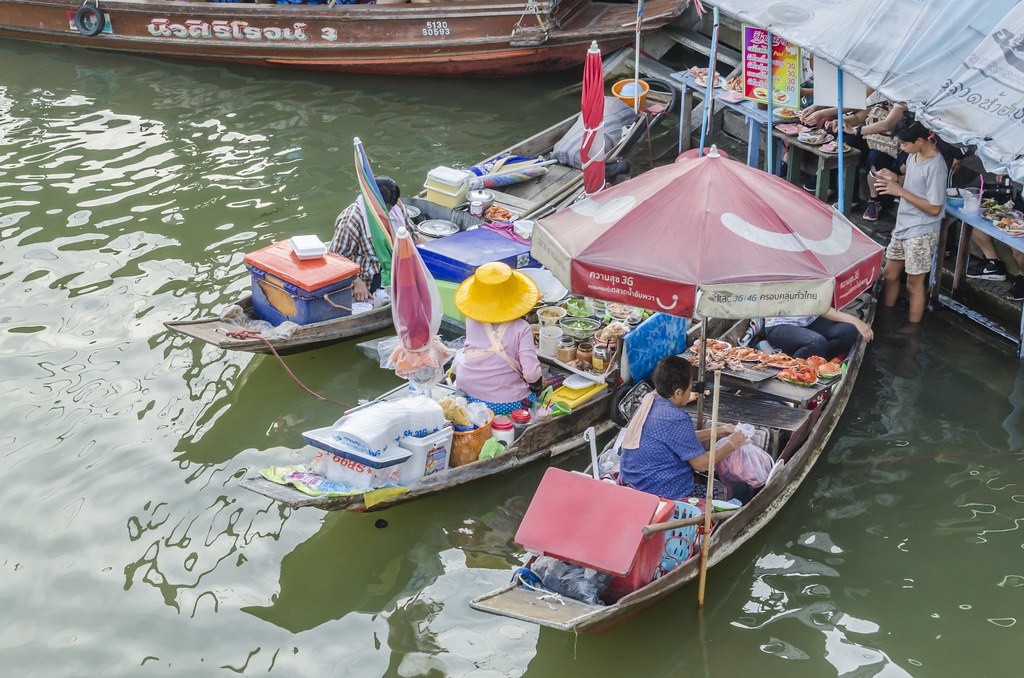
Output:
<box><xmin>696</xmin><ymin>369</ymin><xmax>721</xmax><ymax>607</ymax></box>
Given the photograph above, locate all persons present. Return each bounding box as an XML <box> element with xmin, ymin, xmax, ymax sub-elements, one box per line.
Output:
<box><xmin>454</xmin><ymin>262</ymin><xmax>565</xmax><ymax>414</ymax></box>
<box><xmin>779</xmin><ymin>51</ymin><xmax>1023</xmax><ymax>302</ymax></box>
<box><xmin>765</xmin><ymin>309</ymin><xmax>874</xmax><ymax>361</ymax></box>
<box><xmin>874</xmin><ymin>117</ymin><xmax>949</xmax><ymax>336</ymax></box>
<box><xmin>332</xmin><ymin>176</ymin><xmax>426</xmax><ymax>301</ymax></box>
<box><xmin>619</xmin><ymin>356</ymin><xmax>752</xmax><ymax>503</ymax></box>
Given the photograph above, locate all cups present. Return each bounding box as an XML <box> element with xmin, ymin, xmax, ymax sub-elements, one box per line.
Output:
<box><xmin>963</xmin><ymin>188</ymin><xmax>984</xmax><ymax>218</ymax></box>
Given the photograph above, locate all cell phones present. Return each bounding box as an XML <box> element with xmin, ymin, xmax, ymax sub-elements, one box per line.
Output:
<box><xmin>870</xmin><ymin>170</ymin><xmax>886</xmax><ymax>181</ymax></box>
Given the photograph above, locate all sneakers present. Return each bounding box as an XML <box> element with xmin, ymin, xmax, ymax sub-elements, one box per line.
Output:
<box><xmin>1001</xmin><ymin>273</ymin><xmax>1024</xmax><ymax>300</ymax></box>
<box><xmin>863</xmin><ymin>198</ymin><xmax>882</xmax><ymax>221</ymax></box>
<box><xmin>966</xmin><ymin>255</ymin><xmax>1008</xmax><ymax>281</ymax></box>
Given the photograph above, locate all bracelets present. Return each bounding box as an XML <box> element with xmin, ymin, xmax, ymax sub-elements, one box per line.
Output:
<box><xmin>897</xmin><ymin>176</ymin><xmax>899</xmax><ymax>183</ymax></box>
<box><xmin>857</xmin><ymin>125</ymin><xmax>862</xmax><ymax>136</ymax></box>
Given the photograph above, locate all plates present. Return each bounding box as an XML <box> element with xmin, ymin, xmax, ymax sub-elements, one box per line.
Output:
<box><xmin>712</xmin><ymin>500</ymin><xmax>740</xmax><ymax>511</ymax></box>
<box><xmin>351</xmin><ymin>302</ymin><xmax>373</xmax><ymax>315</ymax></box>
<box><xmin>760</xmin><ymin>355</ymin><xmax>798</xmax><ymax>368</ymax></box>
<box><xmin>818</xmin><ymin>372</ymin><xmax>842</xmax><ymax>379</ymax></box>
<box><xmin>773</xmin><ymin>107</ymin><xmax>801</xmax><ymax>118</ymax></box>
<box><xmin>753</xmin><ymin>87</ymin><xmax>789</xmax><ymax>103</ymax></box>
<box><xmin>691</xmin><ymin>68</ymin><xmax>731</xmax><ymax>90</ymax></box>
<box><xmin>980</xmin><ymin>206</ymin><xmax>1024</xmax><ymax>238</ymax></box>
<box><xmin>729</xmin><ymin>347</ymin><xmax>763</xmax><ymax>361</ymax></box>
<box><xmin>694</xmin><ymin>339</ymin><xmax>732</xmax><ymax>350</ymax></box>
<box><xmin>778</xmin><ymin>372</ymin><xmax>819</xmax><ymax>387</ymax></box>
<box><xmin>718</xmin><ymin>90</ymin><xmax>744</xmax><ymax>104</ymax></box>
<box><xmin>775</xmin><ymin>123</ymin><xmax>851</xmax><ymax>153</ymax></box>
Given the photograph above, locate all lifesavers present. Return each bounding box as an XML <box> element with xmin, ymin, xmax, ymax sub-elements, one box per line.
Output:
<box><xmin>74</xmin><ymin>4</ymin><xmax>105</xmax><ymax>37</ymax></box>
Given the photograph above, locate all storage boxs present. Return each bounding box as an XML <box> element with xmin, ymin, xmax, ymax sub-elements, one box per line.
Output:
<box><xmin>423</xmin><ymin>165</ymin><xmax>470</xmax><ymax>209</ymax></box>
<box><xmin>240</xmin><ymin>239</ymin><xmax>361</xmax><ymax>326</ymax></box>
<box><xmin>416</xmin><ymin>227</ymin><xmax>540</xmax><ymax>323</ymax></box>
<box><xmin>302</xmin><ymin>403</ymin><xmax>455</xmax><ymax>489</ymax></box>
<box><xmin>514</xmin><ymin>467</ymin><xmax>676</xmax><ymax>603</ymax></box>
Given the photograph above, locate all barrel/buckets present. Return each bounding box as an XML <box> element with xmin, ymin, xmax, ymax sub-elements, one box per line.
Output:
<box><xmin>612</xmin><ymin>79</ymin><xmax>650</xmax><ymax>109</ymax></box>
<box><xmin>539</xmin><ymin>326</ymin><xmax>563</xmax><ymax>356</ymax></box>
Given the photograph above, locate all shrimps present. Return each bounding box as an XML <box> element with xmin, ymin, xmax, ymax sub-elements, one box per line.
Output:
<box><xmin>482</xmin><ymin>205</ymin><xmax>512</xmax><ymax>222</ymax></box>
<box><xmin>697</xmin><ymin>338</ymin><xmax>796</xmax><ymax>367</ymax></box>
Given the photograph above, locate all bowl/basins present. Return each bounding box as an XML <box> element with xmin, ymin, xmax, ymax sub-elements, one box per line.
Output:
<box><xmin>514</xmin><ymin>220</ymin><xmax>534</xmax><ymax>239</ymax></box>
<box><xmin>536</xmin><ymin>306</ymin><xmax>567</xmax><ymax>327</ymax></box>
<box><xmin>605</xmin><ymin>301</ymin><xmax>633</xmax><ymax>319</ymax></box>
<box><xmin>945</xmin><ymin>188</ymin><xmax>973</xmax><ymax>208</ymax></box>
<box><xmin>595</xmin><ymin>330</ymin><xmax>616</xmax><ymax>343</ymax></box>
<box><xmin>620</xmin><ymin>82</ymin><xmax>645</xmax><ymax>96</ymax></box>
<box><xmin>559</xmin><ymin>317</ymin><xmax>601</xmax><ymax>340</ymax></box>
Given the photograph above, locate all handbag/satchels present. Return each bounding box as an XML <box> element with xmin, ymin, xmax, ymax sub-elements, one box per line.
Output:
<box><xmin>715</xmin><ymin>421</ymin><xmax>771</xmax><ymax>487</ymax></box>
<box><xmin>864</xmin><ymin>105</ymin><xmax>904</xmax><ymax>160</ymax></box>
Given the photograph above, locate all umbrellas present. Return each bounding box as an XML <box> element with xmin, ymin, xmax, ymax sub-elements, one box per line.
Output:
<box><xmin>580</xmin><ymin>41</ymin><xmax>605</xmax><ymax>196</ymax></box>
<box><xmin>353</xmin><ymin>137</ymin><xmax>396</xmax><ymax>289</ymax></box>
<box><xmin>532</xmin><ymin>145</ymin><xmax>884</xmax><ymax>437</ymax></box>
<box><xmin>390</xmin><ymin>228</ymin><xmax>452</xmax><ymax>399</ymax></box>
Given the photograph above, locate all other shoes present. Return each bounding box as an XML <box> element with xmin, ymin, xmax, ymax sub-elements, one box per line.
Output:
<box><xmin>779</xmin><ymin>161</ymin><xmax>788</xmax><ymax>178</ymax></box>
<box><xmin>803</xmin><ymin>175</ymin><xmax>817</xmax><ymax>191</ymax></box>
<box><xmin>834</xmin><ymin>200</ymin><xmax>858</xmax><ymax>210</ymax></box>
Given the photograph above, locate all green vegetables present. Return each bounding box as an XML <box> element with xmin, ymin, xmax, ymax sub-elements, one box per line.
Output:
<box><xmin>567</xmin><ymin>301</ymin><xmax>595</xmax><ymax>339</ymax></box>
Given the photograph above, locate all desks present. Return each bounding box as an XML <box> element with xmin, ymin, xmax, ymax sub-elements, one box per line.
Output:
<box><xmin>672</xmin><ymin>66</ymin><xmax>1023</xmax><ymax>359</ymax></box>
<box><xmin>675</xmin><ymin>345</ymin><xmax>843</xmax><ymax>409</ymax></box>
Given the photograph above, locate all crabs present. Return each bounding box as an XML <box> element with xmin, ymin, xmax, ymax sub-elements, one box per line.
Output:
<box><xmin>795</xmin><ymin>355</ymin><xmax>841</xmax><ymax>376</ymax></box>
<box><xmin>772</xmin><ymin>364</ymin><xmax>817</xmax><ymax>384</ymax></box>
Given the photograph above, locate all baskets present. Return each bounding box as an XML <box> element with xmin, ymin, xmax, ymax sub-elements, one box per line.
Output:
<box><xmin>449</xmin><ymin>407</ymin><xmax>495</xmax><ymax>467</ymax></box>
<box><xmin>661</xmin><ymin>499</ymin><xmax>702</xmax><ymax>571</ymax></box>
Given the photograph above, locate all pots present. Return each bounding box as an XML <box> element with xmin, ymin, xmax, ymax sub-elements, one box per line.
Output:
<box><xmin>466</xmin><ymin>189</ymin><xmax>496</xmax><ymax>212</ymax></box>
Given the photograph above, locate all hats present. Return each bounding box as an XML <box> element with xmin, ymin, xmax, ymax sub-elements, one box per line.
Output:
<box><xmin>454</xmin><ymin>261</ymin><xmax>539</xmax><ymax>324</ymax></box>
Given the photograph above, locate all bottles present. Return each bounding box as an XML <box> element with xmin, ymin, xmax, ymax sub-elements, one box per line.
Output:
<box><xmin>470</xmin><ymin>202</ymin><xmax>483</xmax><ymax>218</ymax></box>
<box><xmin>511</xmin><ymin>409</ymin><xmax>532</xmax><ymax>441</ymax></box>
<box><xmin>556</xmin><ymin>336</ymin><xmax>612</xmax><ymax>373</ymax></box>
<box><xmin>491</xmin><ymin>418</ymin><xmax>515</xmax><ymax>448</ymax></box>
